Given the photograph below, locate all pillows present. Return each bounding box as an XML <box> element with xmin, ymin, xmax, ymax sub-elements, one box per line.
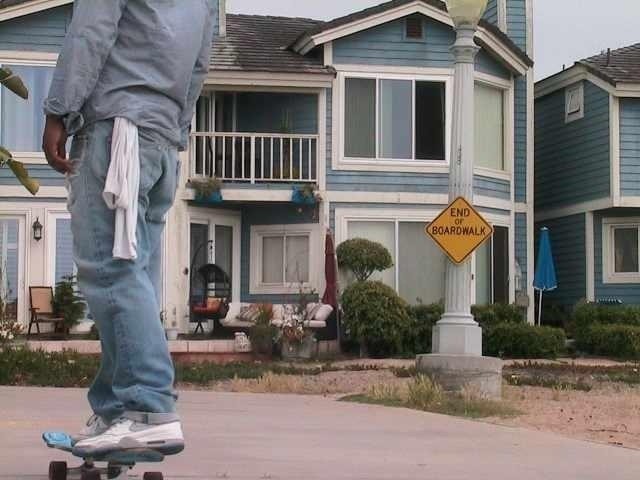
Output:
<box><xmin>305</xmin><ymin>302</ymin><xmax>331</xmax><ymax>320</ymax></box>
<box><xmin>237</xmin><ymin>306</ymin><xmax>256</xmax><ymax>323</ymax></box>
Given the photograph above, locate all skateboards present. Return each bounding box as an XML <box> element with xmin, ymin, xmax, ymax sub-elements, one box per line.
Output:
<box><xmin>43</xmin><ymin>432</ymin><xmax>163</xmax><ymax>480</ymax></box>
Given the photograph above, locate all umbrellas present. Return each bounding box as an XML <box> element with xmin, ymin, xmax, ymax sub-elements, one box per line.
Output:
<box><xmin>321</xmin><ymin>229</ymin><xmax>341</xmax><ymax>350</ymax></box>
<box><xmin>533</xmin><ymin>226</ymin><xmax>558</xmax><ymax>329</ymax></box>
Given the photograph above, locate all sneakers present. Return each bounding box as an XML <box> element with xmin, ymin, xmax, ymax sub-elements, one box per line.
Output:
<box><xmin>71</xmin><ymin>414</ymin><xmax>185</xmax><ymax>458</ymax></box>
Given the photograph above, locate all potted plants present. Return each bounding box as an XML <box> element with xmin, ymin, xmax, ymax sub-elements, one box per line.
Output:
<box><xmin>292</xmin><ymin>182</ymin><xmax>321</xmax><ymax>206</ymax></box>
<box><xmin>193</xmin><ymin>177</ymin><xmax>224</xmax><ymax>203</ymax></box>
<box><xmin>279</xmin><ymin>281</ymin><xmax>323</xmax><ymax>361</ymax></box>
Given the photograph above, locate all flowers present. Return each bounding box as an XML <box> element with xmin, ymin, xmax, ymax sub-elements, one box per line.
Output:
<box><xmin>253</xmin><ymin>304</ymin><xmax>274</xmax><ymax>324</ymax></box>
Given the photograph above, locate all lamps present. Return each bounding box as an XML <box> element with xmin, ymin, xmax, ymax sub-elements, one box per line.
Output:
<box><xmin>32</xmin><ymin>217</ymin><xmax>42</xmax><ymax>241</ymax></box>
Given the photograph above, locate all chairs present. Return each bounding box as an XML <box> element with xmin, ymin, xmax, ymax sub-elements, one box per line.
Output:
<box><xmin>191</xmin><ymin>265</ymin><xmax>231</xmax><ymax>319</ymax></box>
<box><xmin>29</xmin><ymin>286</ymin><xmax>67</xmax><ymax>341</ymax></box>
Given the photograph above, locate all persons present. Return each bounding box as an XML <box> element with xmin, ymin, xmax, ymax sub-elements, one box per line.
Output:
<box><xmin>43</xmin><ymin>0</ymin><xmax>212</xmax><ymax>457</ymax></box>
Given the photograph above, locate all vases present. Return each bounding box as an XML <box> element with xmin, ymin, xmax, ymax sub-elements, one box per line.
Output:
<box><xmin>248</xmin><ymin>325</ymin><xmax>277</xmax><ymax>354</ymax></box>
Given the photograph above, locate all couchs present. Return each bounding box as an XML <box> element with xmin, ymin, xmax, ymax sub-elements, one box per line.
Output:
<box><xmin>221</xmin><ymin>304</ymin><xmax>334</xmax><ymax>342</ymax></box>
